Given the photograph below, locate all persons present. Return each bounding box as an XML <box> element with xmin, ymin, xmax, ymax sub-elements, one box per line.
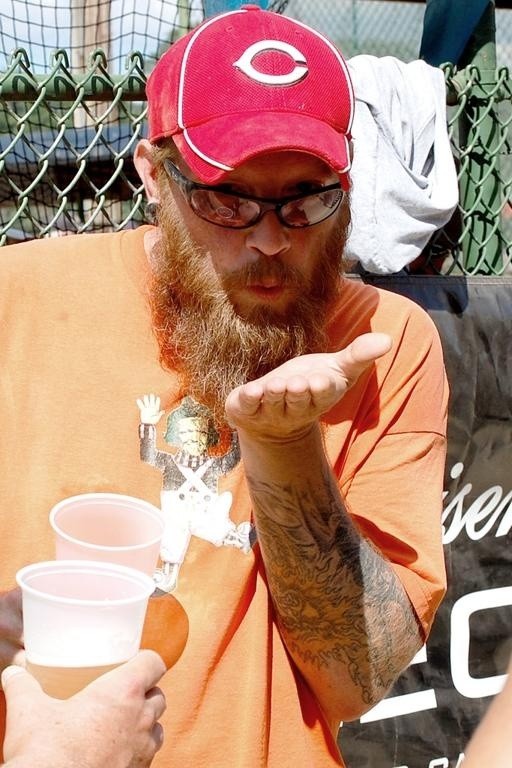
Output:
<box><xmin>134</xmin><ymin>393</ymin><xmax>258</xmax><ymax>601</ymax></box>
<box><xmin>452</xmin><ymin>667</ymin><xmax>512</xmax><ymax>768</ymax></box>
<box><xmin>1</xmin><ymin>4</ymin><xmax>452</xmax><ymax>768</ymax></box>
<box><xmin>1</xmin><ymin>648</ymin><xmax>171</xmax><ymax>768</ymax></box>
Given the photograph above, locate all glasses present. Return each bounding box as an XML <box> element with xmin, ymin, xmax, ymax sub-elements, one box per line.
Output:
<box><xmin>163</xmin><ymin>158</ymin><xmax>346</xmax><ymax>229</ymax></box>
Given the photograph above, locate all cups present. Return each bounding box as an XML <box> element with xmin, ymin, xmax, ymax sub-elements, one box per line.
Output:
<box><xmin>16</xmin><ymin>560</ymin><xmax>155</xmax><ymax>699</ymax></box>
<box><xmin>49</xmin><ymin>493</ymin><xmax>168</xmax><ymax>607</ymax></box>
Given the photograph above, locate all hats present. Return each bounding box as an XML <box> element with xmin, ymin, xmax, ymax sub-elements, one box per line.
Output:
<box><xmin>146</xmin><ymin>4</ymin><xmax>355</xmax><ymax>185</ymax></box>
